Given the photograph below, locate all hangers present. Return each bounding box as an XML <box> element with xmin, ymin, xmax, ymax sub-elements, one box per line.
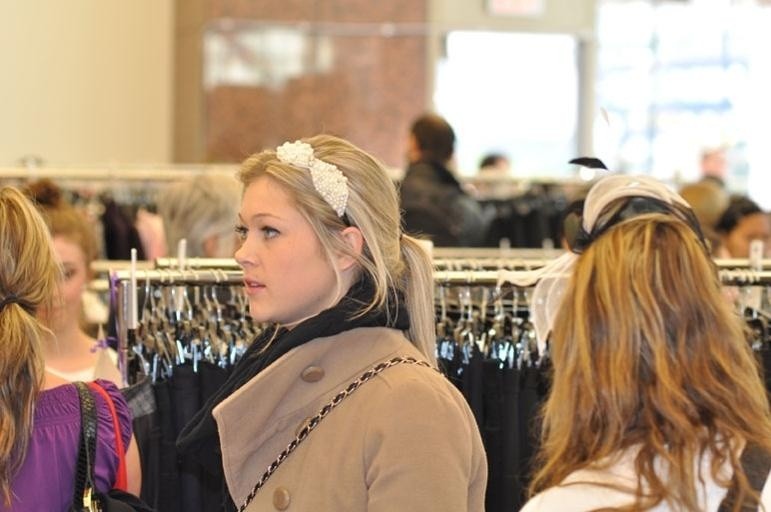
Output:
<box><xmin>427</xmin><ymin>280</ymin><xmax>551</xmax><ymax>375</ymax></box>
<box><xmin>719</xmin><ymin>268</ymin><xmax>767</xmax><ymax>340</ymax></box>
<box><xmin>126</xmin><ymin>270</ymin><xmax>254</xmax><ymax>388</ymax></box>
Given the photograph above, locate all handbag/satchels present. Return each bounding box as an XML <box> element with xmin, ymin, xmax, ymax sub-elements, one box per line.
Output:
<box><xmin>71</xmin><ymin>381</ymin><xmax>152</xmax><ymax>512</ymax></box>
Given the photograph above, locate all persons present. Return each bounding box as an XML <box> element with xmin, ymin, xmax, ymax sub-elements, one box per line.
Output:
<box><xmin>518</xmin><ymin>211</ymin><xmax>771</xmax><ymax>512</ymax></box>
<box><xmin>401</xmin><ymin>113</ymin><xmax>770</xmax><ymax>259</ymax></box>
<box><xmin>0</xmin><ymin>185</ymin><xmax>143</xmax><ymax>512</ymax></box>
<box><xmin>174</xmin><ymin>133</ymin><xmax>490</xmax><ymax>512</ymax></box>
<box><xmin>37</xmin><ymin>213</ymin><xmax>124</xmax><ymax>391</ymax></box>
